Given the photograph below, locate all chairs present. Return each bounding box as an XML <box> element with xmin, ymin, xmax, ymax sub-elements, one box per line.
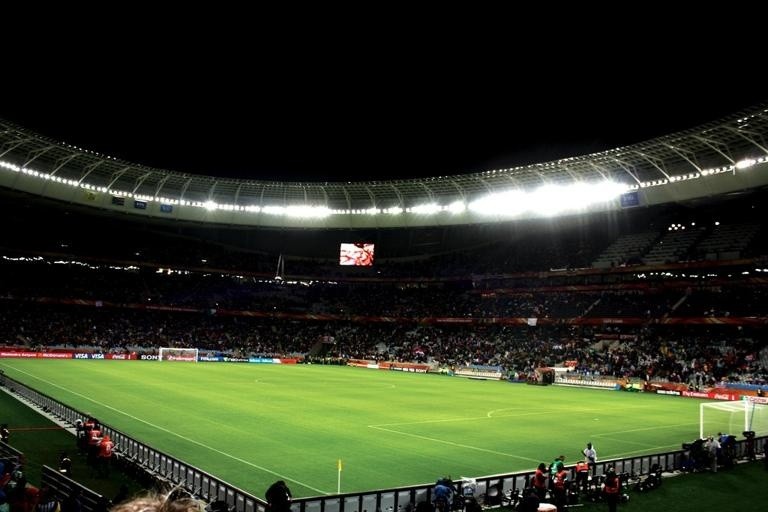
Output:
<box><xmin>392</xmin><ymin>468</ymin><xmax>633</xmax><ymax>512</ymax></box>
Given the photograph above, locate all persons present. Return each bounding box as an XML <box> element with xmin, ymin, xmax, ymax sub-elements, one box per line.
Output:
<box><xmin>0</xmin><ymin>417</ymin><xmax>292</xmax><ymax>511</ymax></box>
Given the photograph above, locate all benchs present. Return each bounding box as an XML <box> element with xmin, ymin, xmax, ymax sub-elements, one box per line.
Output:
<box><xmin>590</xmin><ymin>192</ymin><xmax>766</xmax><ymax>269</ymax></box>
<box><xmin>1</xmin><ymin>432</ymin><xmax>124</xmax><ymax>511</ymax></box>
